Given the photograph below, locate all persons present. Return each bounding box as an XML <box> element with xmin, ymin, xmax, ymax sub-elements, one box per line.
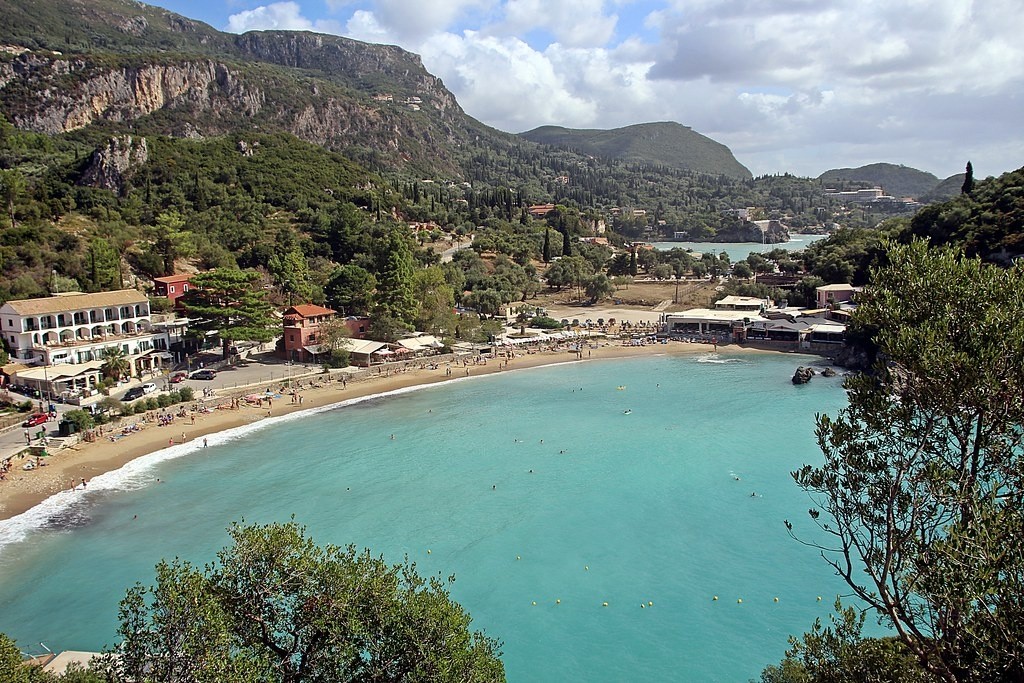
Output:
<box><xmin>0</xmin><ymin>318</ymin><xmax>756</xmax><ymax>525</ymax></box>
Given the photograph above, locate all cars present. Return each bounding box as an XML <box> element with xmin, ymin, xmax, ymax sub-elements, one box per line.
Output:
<box><xmin>26</xmin><ymin>413</ymin><xmax>48</xmax><ymax>428</ymax></box>
<box><xmin>142</xmin><ymin>382</ymin><xmax>157</xmax><ymax>394</ymax></box>
<box><xmin>124</xmin><ymin>387</ymin><xmax>145</xmax><ymax>401</ymax></box>
<box><xmin>190</xmin><ymin>369</ymin><xmax>217</xmax><ymax>381</ymax></box>
<box><xmin>169</xmin><ymin>371</ymin><xmax>186</xmax><ymax>384</ymax></box>
